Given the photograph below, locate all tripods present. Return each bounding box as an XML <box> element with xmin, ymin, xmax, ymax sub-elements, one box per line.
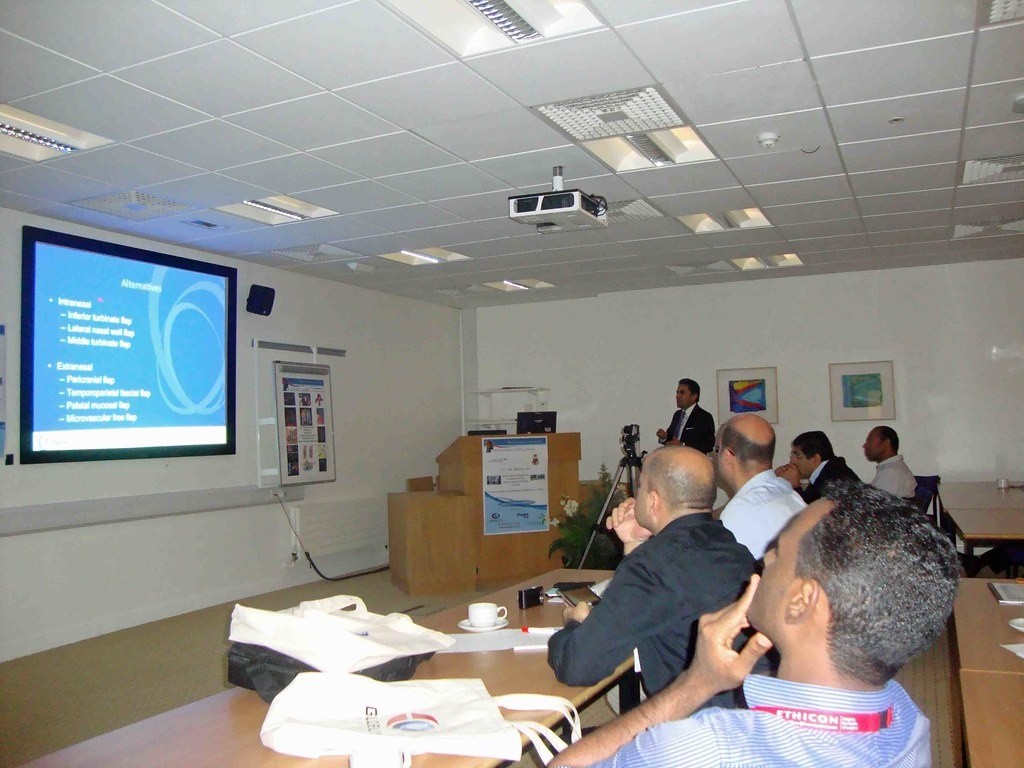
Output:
<box><xmin>579</xmin><ymin>436</ymin><xmax>648</xmax><ymax>570</ymax></box>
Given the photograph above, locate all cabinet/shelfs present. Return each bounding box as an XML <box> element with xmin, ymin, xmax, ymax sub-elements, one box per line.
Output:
<box><xmin>467</xmin><ymin>387</ymin><xmax>550</xmax><ymax>430</ymax></box>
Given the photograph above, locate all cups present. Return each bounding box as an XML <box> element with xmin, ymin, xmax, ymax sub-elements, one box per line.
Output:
<box><xmin>348</xmin><ymin>735</ymin><xmax>412</xmax><ymax>768</ymax></box>
<box><xmin>468</xmin><ymin>602</ymin><xmax>507</xmax><ymax>628</ymax></box>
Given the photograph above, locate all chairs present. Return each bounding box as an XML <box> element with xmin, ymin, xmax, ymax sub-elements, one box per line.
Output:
<box><xmin>909</xmin><ymin>475</ymin><xmax>941</xmax><ymax>523</ymax></box>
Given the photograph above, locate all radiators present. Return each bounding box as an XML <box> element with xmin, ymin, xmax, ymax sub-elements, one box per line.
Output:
<box><xmin>289</xmin><ymin>496</ymin><xmax>389</xmax><ymax>566</ymax></box>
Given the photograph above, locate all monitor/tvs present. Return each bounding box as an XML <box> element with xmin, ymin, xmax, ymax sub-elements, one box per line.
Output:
<box><xmin>516</xmin><ymin>412</ymin><xmax>557</xmax><ymax>435</ymax></box>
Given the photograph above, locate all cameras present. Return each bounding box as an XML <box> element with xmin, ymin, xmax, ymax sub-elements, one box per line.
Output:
<box><xmin>624</xmin><ymin>424</ymin><xmax>640</xmax><ymax>436</ymax></box>
<box><xmin>517</xmin><ymin>586</ymin><xmax>544</xmax><ymax>610</ymax></box>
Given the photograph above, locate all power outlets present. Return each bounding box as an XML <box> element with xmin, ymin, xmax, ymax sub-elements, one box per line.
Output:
<box><xmin>270</xmin><ymin>489</ymin><xmax>283</xmax><ymax>500</ymax></box>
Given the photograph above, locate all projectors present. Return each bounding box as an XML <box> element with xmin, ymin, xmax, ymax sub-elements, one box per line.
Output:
<box><xmin>510</xmin><ymin>191</ymin><xmax>606</xmax><ymax>233</ymax></box>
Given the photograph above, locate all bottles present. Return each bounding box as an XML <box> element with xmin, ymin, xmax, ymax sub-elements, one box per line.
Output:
<box><xmin>996</xmin><ymin>454</ymin><xmax>1009</xmax><ymax>489</ymax></box>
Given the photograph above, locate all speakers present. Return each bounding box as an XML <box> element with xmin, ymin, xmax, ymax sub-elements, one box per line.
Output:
<box><xmin>246</xmin><ymin>285</ymin><xmax>275</xmax><ymax>316</ymax></box>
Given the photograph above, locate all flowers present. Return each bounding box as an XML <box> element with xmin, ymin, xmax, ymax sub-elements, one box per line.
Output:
<box><xmin>544</xmin><ymin>463</ymin><xmax>628</xmax><ymax>569</ymax></box>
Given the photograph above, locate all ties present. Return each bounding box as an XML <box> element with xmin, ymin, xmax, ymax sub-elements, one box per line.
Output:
<box><xmin>674</xmin><ymin>412</ymin><xmax>685</xmax><ymax>440</ymax></box>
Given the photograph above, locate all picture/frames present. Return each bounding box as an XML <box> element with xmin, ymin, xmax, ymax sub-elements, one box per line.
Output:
<box><xmin>829</xmin><ymin>361</ymin><xmax>896</xmax><ymax>422</ymax></box>
<box><xmin>717</xmin><ymin>367</ymin><xmax>778</xmax><ymax>425</ymax></box>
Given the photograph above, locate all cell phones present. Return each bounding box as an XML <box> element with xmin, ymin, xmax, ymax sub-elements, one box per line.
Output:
<box><xmin>558</xmin><ymin>587</ymin><xmax>601</xmax><ymax>610</ymax></box>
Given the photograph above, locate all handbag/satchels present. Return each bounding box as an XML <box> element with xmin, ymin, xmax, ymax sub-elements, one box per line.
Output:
<box><xmin>229</xmin><ymin>595</ymin><xmax>458</xmax><ymax>676</ymax></box>
<box><xmin>227</xmin><ymin>639</ymin><xmax>437</xmax><ymax>707</ymax></box>
<box><xmin>260</xmin><ymin>669</ymin><xmax>582</xmax><ymax>768</ymax></box>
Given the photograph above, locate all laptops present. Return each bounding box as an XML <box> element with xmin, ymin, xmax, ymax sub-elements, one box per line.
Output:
<box><xmin>468</xmin><ymin>430</ymin><xmax>506</xmax><ymax>436</ymax></box>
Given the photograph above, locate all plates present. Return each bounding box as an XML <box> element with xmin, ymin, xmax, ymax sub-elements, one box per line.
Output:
<box><xmin>1008</xmin><ymin>618</ymin><xmax>1024</xmax><ymax>631</ymax></box>
<box><xmin>457</xmin><ymin>618</ymin><xmax>509</xmax><ymax>633</ymax></box>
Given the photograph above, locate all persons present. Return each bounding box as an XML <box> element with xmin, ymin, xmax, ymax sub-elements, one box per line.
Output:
<box><xmin>775</xmin><ymin>431</ymin><xmax>867</xmax><ymax>505</ymax></box>
<box><xmin>711</xmin><ymin>414</ymin><xmax>809</xmax><ymax>561</ymax></box>
<box><xmin>860</xmin><ymin>426</ymin><xmax>918</xmax><ymax>499</ymax></box>
<box><xmin>547</xmin><ymin>446</ymin><xmax>781</xmax><ymax>710</ymax></box>
<box><xmin>546</xmin><ymin>480</ymin><xmax>960</xmax><ymax>768</ymax></box>
<box><xmin>656</xmin><ymin>378</ymin><xmax>715</xmax><ymax>456</ymax></box>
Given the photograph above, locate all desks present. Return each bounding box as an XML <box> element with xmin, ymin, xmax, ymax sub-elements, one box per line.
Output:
<box><xmin>946</xmin><ymin>509</ymin><xmax>1024</xmax><ymax>578</ymax></box>
<box><xmin>954</xmin><ymin>579</ymin><xmax>1024</xmax><ymax>676</ymax></box>
<box><xmin>937</xmin><ymin>482</ymin><xmax>1024</xmax><ymax>532</ymax></box>
<box><xmin>18</xmin><ymin>568</ymin><xmax>640</xmax><ymax>767</ymax></box>
<box><xmin>959</xmin><ymin>670</ymin><xmax>1024</xmax><ymax>768</ymax></box>
